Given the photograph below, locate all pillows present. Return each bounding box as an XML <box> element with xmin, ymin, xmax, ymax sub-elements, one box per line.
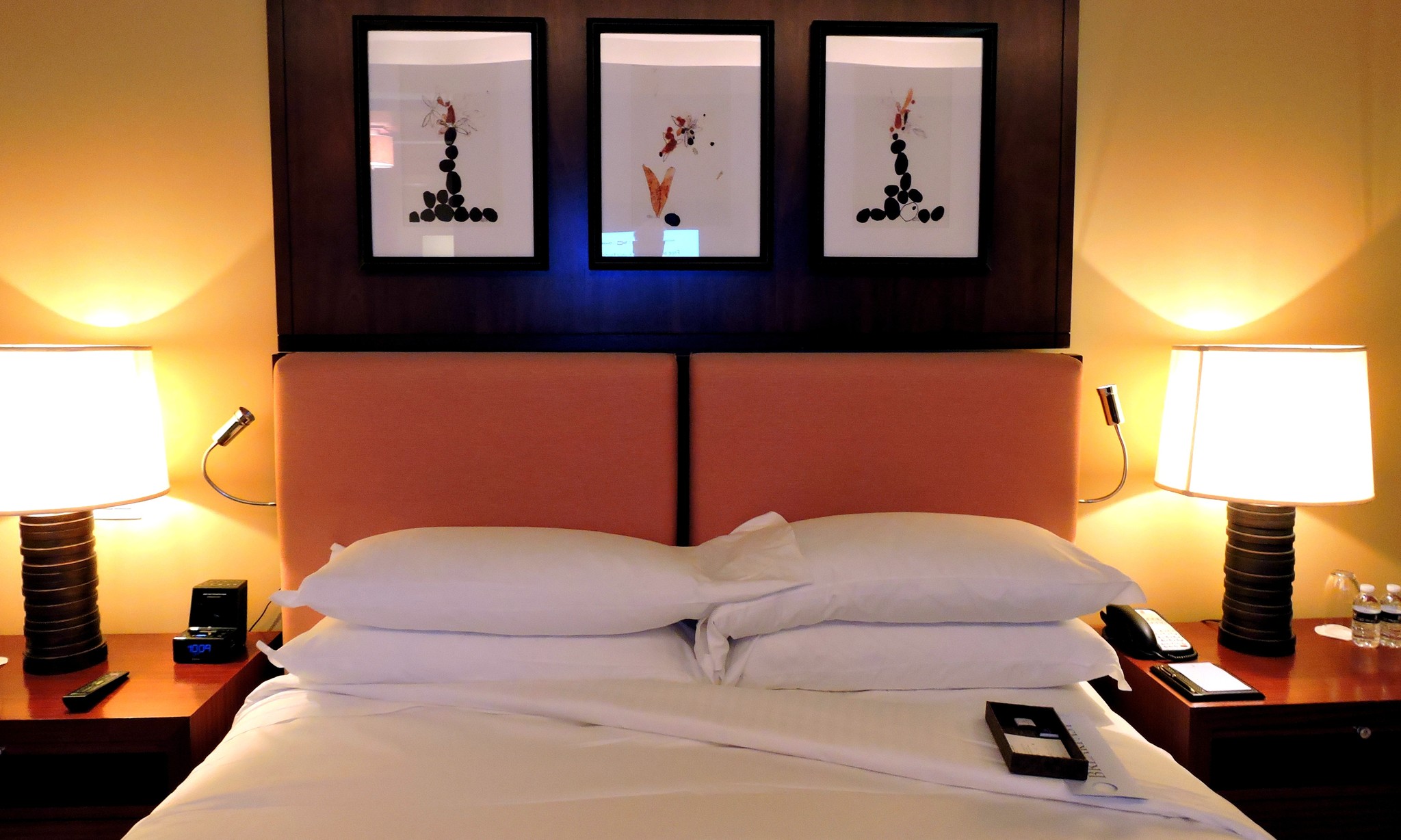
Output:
<box><xmin>695</xmin><ymin>512</ymin><xmax>1148</xmax><ymax>682</ymax></box>
<box><xmin>256</xmin><ymin>615</ymin><xmax>706</xmax><ymax>685</ymax></box>
<box><xmin>271</xmin><ymin>510</ymin><xmax>812</xmax><ymax>635</ymax></box>
<box><xmin>717</xmin><ymin>617</ymin><xmax>1133</xmax><ymax>692</ymax></box>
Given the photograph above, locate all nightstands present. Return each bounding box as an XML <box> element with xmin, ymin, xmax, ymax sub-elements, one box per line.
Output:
<box><xmin>1088</xmin><ymin>615</ymin><xmax>1401</xmax><ymax>840</ymax></box>
<box><xmin>0</xmin><ymin>631</ymin><xmax>279</xmax><ymax>840</ymax></box>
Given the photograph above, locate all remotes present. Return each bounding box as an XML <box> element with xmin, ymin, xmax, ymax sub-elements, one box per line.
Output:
<box><xmin>60</xmin><ymin>669</ymin><xmax>130</xmax><ymax>711</ymax></box>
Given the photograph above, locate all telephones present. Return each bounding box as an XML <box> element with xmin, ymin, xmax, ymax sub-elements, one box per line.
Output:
<box><xmin>1100</xmin><ymin>604</ymin><xmax>1198</xmax><ymax>661</ymax></box>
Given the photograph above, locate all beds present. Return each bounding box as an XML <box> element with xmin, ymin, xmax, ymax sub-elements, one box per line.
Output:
<box><xmin>122</xmin><ymin>353</ymin><xmax>1277</xmax><ymax>840</ymax></box>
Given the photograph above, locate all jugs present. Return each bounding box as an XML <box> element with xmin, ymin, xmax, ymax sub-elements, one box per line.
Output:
<box><xmin>1313</xmin><ymin>570</ymin><xmax>1360</xmax><ymax>641</ymax></box>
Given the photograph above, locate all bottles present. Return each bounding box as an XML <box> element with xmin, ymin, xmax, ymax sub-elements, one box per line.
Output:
<box><xmin>1351</xmin><ymin>584</ymin><xmax>1382</xmax><ymax>648</ymax></box>
<box><xmin>1380</xmin><ymin>584</ymin><xmax>1401</xmax><ymax>648</ymax></box>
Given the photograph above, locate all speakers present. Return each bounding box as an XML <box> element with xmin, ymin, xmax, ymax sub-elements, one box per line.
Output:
<box><xmin>187</xmin><ymin>578</ymin><xmax>247</xmax><ymax>654</ymax></box>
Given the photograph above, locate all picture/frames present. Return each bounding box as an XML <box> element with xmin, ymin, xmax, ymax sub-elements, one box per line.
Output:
<box><xmin>351</xmin><ymin>13</ymin><xmax>550</xmax><ymax>272</ymax></box>
<box><xmin>585</xmin><ymin>17</ymin><xmax>775</xmax><ymax>271</ymax></box>
<box><xmin>810</xmin><ymin>18</ymin><xmax>998</xmax><ymax>271</ymax></box>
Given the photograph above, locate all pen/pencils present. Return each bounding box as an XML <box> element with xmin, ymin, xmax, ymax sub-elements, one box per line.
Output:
<box><xmin>1159</xmin><ymin>664</ymin><xmax>1196</xmax><ymax>693</ymax></box>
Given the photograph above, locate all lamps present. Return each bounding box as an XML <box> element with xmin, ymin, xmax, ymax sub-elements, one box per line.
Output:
<box><xmin>1078</xmin><ymin>384</ymin><xmax>1124</xmax><ymax>503</ymax></box>
<box><xmin>0</xmin><ymin>344</ymin><xmax>171</xmax><ymax>675</ymax></box>
<box><xmin>201</xmin><ymin>407</ymin><xmax>275</xmax><ymax>507</ymax></box>
<box><xmin>1154</xmin><ymin>343</ymin><xmax>1374</xmax><ymax>660</ymax></box>
